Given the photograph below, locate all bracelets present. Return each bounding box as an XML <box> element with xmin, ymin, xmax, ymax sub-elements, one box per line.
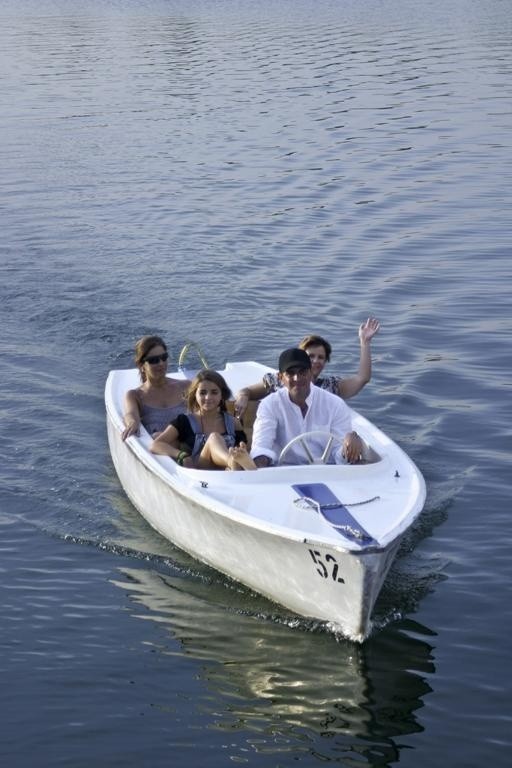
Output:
<box><xmin>177</xmin><ymin>450</ymin><xmax>190</xmax><ymax>466</ymax></box>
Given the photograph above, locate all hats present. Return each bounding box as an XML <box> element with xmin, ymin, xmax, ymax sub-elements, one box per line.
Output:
<box><xmin>279</xmin><ymin>349</ymin><xmax>311</xmax><ymax>372</ymax></box>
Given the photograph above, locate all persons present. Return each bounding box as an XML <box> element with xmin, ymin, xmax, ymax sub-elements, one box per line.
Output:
<box><xmin>248</xmin><ymin>347</ymin><xmax>368</xmax><ymax>468</ymax></box>
<box><xmin>234</xmin><ymin>315</ymin><xmax>382</xmax><ymax>421</ymax></box>
<box><xmin>149</xmin><ymin>369</ymin><xmax>258</xmax><ymax>473</ymax></box>
<box><xmin>121</xmin><ymin>334</ymin><xmax>195</xmax><ymax>446</ymax></box>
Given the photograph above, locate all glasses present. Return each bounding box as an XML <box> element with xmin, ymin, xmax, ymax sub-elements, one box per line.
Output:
<box><xmin>141</xmin><ymin>352</ymin><xmax>168</xmax><ymax>363</ymax></box>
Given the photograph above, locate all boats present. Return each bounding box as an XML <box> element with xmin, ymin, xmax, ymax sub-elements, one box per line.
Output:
<box><xmin>102</xmin><ymin>356</ymin><xmax>429</xmax><ymax>640</ymax></box>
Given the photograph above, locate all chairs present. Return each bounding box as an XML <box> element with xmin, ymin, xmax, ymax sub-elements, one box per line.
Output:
<box><xmin>225</xmin><ymin>396</ymin><xmax>265</xmax><ymax>452</ymax></box>
